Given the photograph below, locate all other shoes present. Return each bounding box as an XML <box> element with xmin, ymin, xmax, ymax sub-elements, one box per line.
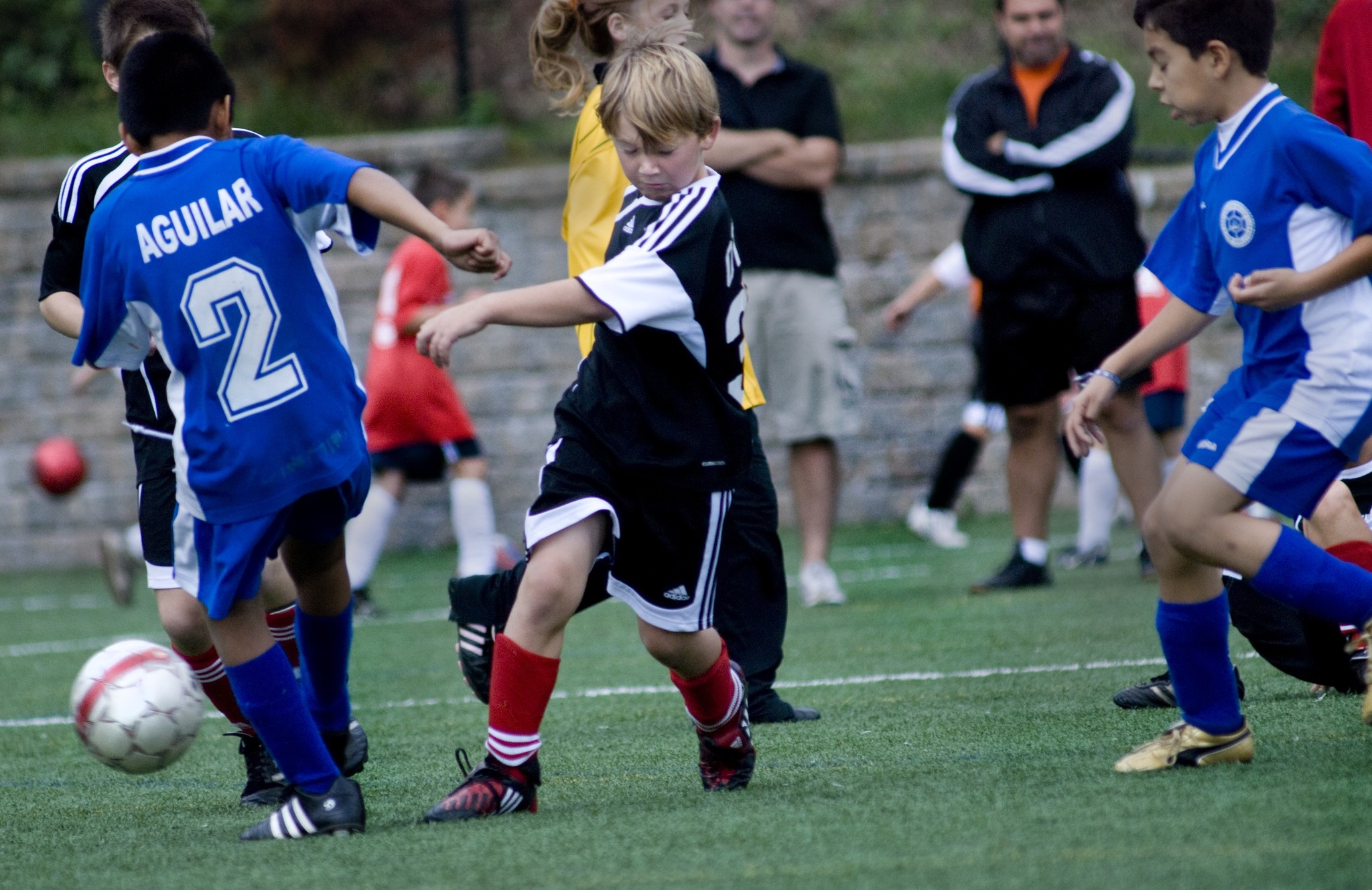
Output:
<box><xmin>351</xmin><ymin>587</ymin><xmax>382</xmax><ymax>617</ymax></box>
<box><xmin>1114</xmin><ymin>493</ymin><xmax>1138</xmax><ymax>526</ymax></box>
<box><xmin>1055</xmin><ymin>541</ymin><xmax>1110</xmax><ymax>569</ymax></box>
<box><xmin>908</xmin><ymin>499</ymin><xmax>971</xmax><ymax>549</ymax></box>
<box><xmin>798</xmin><ymin>558</ymin><xmax>846</xmax><ymax>607</ymax></box>
<box><xmin>100</xmin><ymin>529</ymin><xmax>140</xmax><ymax>606</ymax></box>
<box><xmin>1136</xmin><ymin>547</ymin><xmax>1160</xmax><ymax>583</ymax></box>
<box><xmin>971</xmin><ymin>541</ymin><xmax>1055</xmax><ymax>597</ymax></box>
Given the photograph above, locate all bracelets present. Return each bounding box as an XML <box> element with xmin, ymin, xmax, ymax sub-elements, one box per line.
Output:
<box><xmin>1073</xmin><ymin>370</ymin><xmax>1123</xmax><ymax>392</ymax></box>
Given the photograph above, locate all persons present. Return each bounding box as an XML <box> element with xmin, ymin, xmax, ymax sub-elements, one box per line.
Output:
<box><xmin>696</xmin><ymin>0</ymin><xmax>858</xmax><ymax>608</ymax></box>
<box><xmin>416</xmin><ymin>45</ymin><xmax>761</xmax><ymax>833</ymax></box>
<box><xmin>1053</xmin><ymin>171</ymin><xmax>1188</xmax><ymax>578</ymax></box>
<box><xmin>942</xmin><ymin>1</ymin><xmax>1163</xmax><ymax>585</ymax></box>
<box><xmin>67</xmin><ymin>32</ymin><xmax>510</xmax><ymax>843</ymax></box>
<box><xmin>528</xmin><ymin>0</ymin><xmax>827</xmax><ymax>722</ymax></box>
<box><xmin>345</xmin><ymin>161</ymin><xmax>507</xmax><ymax>628</ymax></box>
<box><xmin>875</xmin><ymin>225</ymin><xmax>1078</xmax><ymax>561</ymax></box>
<box><xmin>1066</xmin><ymin>0</ymin><xmax>1370</xmax><ymax>779</ymax></box>
<box><xmin>36</xmin><ymin>3</ymin><xmax>300</xmax><ymax>808</ymax></box>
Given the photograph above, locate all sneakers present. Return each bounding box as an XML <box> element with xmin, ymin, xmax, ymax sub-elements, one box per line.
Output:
<box><xmin>794</xmin><ymin>706</ymin><xmax>821</xmax><ymax>720</ymax></box>
<box><xmin>424</xmin><ymin>747</ymin><xmax>538</xmax><ymax>821</ymax></box>
<box><xmin>1114</xmin><ymin>713</ymin><xmax>1254</xmax><ymax>773</ymax></box>
<box><xmin>277</xmin><ymin>714</ymin><xmax>368</xmax><ymax>803</ymax></box>
<box><xmin>1345</xmin><ymin>618</ymin><xmax>1372</xmax><ymax>724</ymax></box>
<box><xmin>1114</xmin><ymin>664</ymin><xmax>1244</xmax><ymax>709</ymax></box>
<box><xmin>455</xmin><ymin>619</ymin><xmax>498</xmax><ymax>704</ymax></box>
<box><xmin>696</xmin><ymin>660</ymin><xmax>756</xmax><ymax>793</ymax></box>
<box><xmin>222</xmin><ymin>732</ymin><xmax>292</xmax><ymax>808</ymax></box>
<box><xmin>241</xmin><ymin>778</ymin><xmax>366</xmax><ymax>840</ymax></box>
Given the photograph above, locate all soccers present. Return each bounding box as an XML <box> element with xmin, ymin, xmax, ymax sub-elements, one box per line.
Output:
<box><xmin>32</xmin><ymin>436</ymin><xmax>87</xmax><ymax>496</ymax></box>
<box><xmin>69</xmin><ymin>636</ymin><xmax>205</xmax><ymax>774</ymax></box>
